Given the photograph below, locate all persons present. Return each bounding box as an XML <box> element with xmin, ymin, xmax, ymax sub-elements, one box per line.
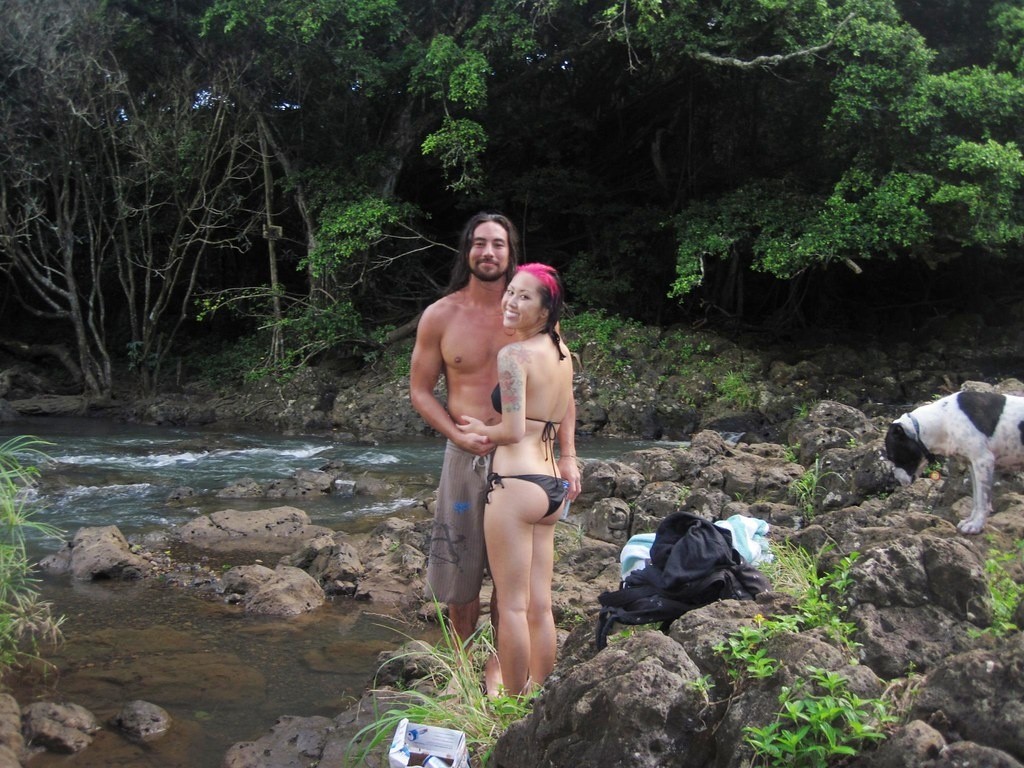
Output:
<box><xmin>454</xmin><ymin>263</ymin><xmax>581</xmax><ymax>713</ymax></box>
<box><xmin>410</xmin><ymin>214</ymin><xmax>562</xmax><ymax>714</ymax></box>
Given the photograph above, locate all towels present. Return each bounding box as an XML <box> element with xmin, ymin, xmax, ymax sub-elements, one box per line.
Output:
<box><xmin>620</xmin><ymin>514</ymin><xmax>775</xmax><ymax>589</ymax></box>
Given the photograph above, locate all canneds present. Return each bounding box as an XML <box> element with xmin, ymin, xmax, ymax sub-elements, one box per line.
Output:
<box><xmin>421</xmin><ymin>755</ymin><xmax>450</xmax><ymax>768</ymax></box>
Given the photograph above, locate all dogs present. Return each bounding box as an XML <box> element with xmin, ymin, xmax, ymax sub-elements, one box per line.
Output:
<box><xmin>885</xmin><ymin>391</ymin><xmax>1024</xmax><ymax>534</ymax></box>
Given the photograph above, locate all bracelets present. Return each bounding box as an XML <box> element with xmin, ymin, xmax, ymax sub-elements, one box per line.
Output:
<box><xmin>561</xmin><ymin>455</ymin><xmax>576</xmax><ymax>458</ymax></box>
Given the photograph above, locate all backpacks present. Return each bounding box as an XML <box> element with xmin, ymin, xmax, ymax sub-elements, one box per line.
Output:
<box><xmin>595</xmin><ymin>512</ymin><xmax>773</xmax><ymax>652</ymax></box>
